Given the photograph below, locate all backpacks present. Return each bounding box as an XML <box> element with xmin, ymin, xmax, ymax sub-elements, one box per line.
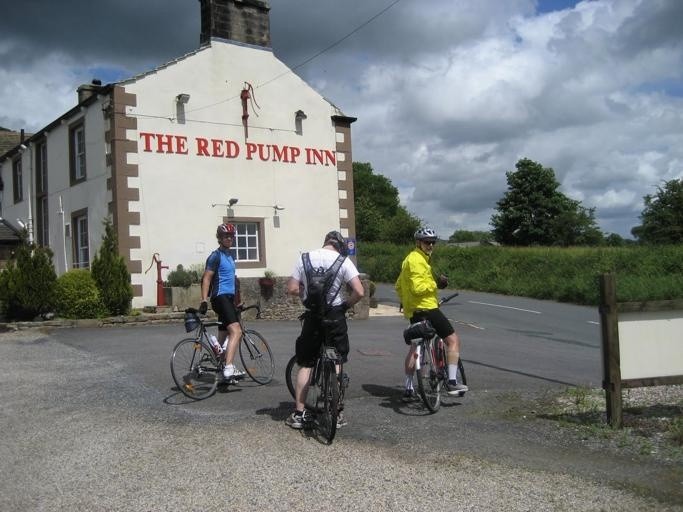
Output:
<box><xmin>302</xmin><ymin>253</ymin><xmax>347</xmax><ymax>310</ymax></box>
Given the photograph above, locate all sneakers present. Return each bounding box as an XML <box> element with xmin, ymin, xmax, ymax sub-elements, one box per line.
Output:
<box><xmin>223</xmin><ymin>364</ymin><xmax>246</xmax><ymax>378</ymax></box>
<box><xmin>336</xmin><ymin>411</ymin><xmax>347</xmax><ymax>429</ymax></box>
<box><xmin>286</xmin><ymin>411</ymin><xmax>304</xmax><ymax>429</ymax></box>
<box><xmin>403</xmin><ymin>390</ymin><xmax>420</xmax><ymax>401</ymax></box>
<box><xmin>448</xmin><ymin>381</ymin><xmax>469</xmax><ymax>395</ymax></box>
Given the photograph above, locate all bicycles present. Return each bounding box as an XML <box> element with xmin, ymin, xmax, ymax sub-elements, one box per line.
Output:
<box><xmin>285</xmin><ymin>311</ymin><xmax>355</xmax><ymax>445</ymax></box>
<box><xmin>400</xmin><ymin>293</ymin><xmax>467</xmax><ymax>413</ymax></box>
<box><xmin>170</xmin><ymin>303</ymin><xmax>274</xmax><ymax>400</ymax></box>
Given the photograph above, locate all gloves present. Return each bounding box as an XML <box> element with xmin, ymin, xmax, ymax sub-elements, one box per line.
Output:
<box><xmin>437</xmin><ymin>274</ymin><xmax>448</xmax><ymax>289</ymax></box>
<box><xmin>199</xmin><ymin>300</ymin><xmax>208</xmax><ymax>315</ymax></box>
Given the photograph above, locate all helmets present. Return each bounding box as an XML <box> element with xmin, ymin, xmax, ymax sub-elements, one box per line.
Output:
<box><xmin>414</xmin><ymin>226</ymin><xmax>437</xmax><ymax>239</ymax></box>
<box><xmin>217</xmin><ymin>222</ymin><xmax>237</xmax><ymax>235</ymax></box>
<box><xmin>325</xmin><ymin>231</ymin><xmax>345</xmax><ymax>247</ymax></box>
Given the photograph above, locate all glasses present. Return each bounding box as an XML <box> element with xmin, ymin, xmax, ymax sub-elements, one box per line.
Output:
<box><xmin>420</xmin><ymin>240</ymin><xmax>436</xmax><ymax>245</ymax></box>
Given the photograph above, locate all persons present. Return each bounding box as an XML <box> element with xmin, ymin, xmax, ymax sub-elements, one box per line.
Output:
<box><xmin>199</xmin><ymin>222</ymin><xmax>246</xmax><ymax>386</ymax></box>
<box><xmin>286</xmin><ymin>231</ymin><xmax>365</xmax><ymax>430</ymax></box>
<box><xmin>394</xmin><ymin>227</ymin><xmax>468</xmax><ymax>402</ymax></box>
